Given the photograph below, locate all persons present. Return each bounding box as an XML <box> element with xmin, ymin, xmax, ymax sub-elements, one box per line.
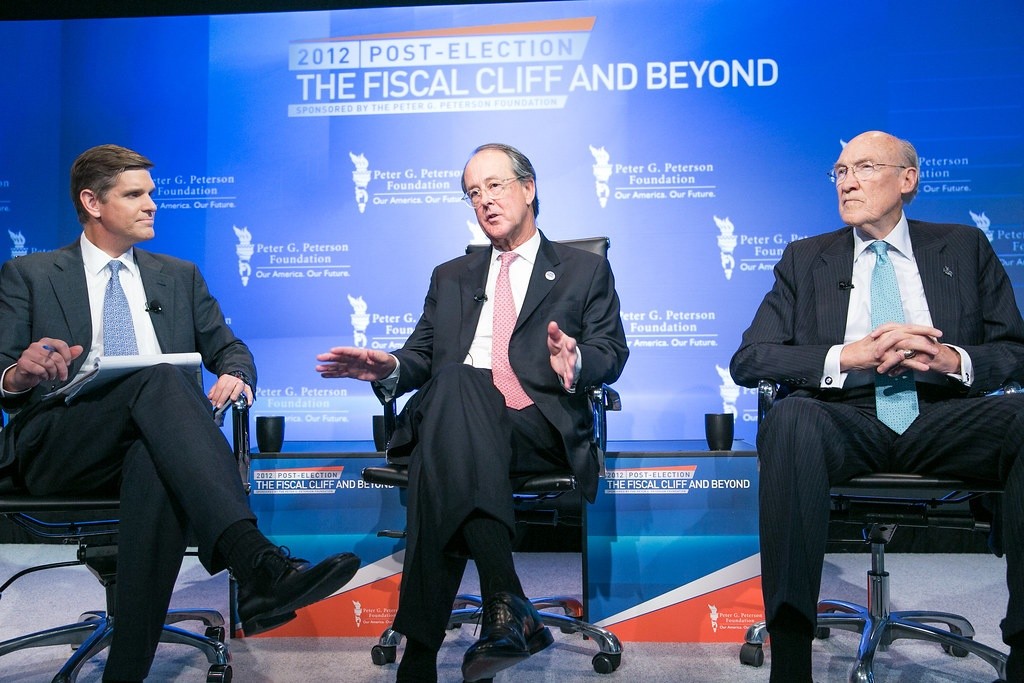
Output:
<box><xmin>0</xmin><ymin>145</ymin><xmax>362</xmax><ymax>683</ymax></box>
<box><xmin>730</xmin><ymin>129</ymin><xmax>1024</xmax><ymax>683</ymax></box>
<box><xmin>315</xmin><ymin>142</ymin><xmax>630</xmax><ymax>683</ymax></box>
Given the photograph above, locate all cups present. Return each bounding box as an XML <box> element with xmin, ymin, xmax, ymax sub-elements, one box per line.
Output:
<box><xmin>705</xmin><ymin>413</ymin><xmax>734</xmax><ymax>451</ymax></box>
<box><xmin>372</xmin><ymin>415</ymin><xmax>398</xmax><ymax>452</ymax></box>
<box><xmin>256</xmin><ymin>416</ymin><xmax>285</xmax><ymax>453</ymax></box>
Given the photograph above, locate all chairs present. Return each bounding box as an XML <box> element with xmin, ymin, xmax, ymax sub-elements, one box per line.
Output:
<box><xmin>0</xmin><ymin>364</ymin><xmax>253</xmax><ymax>683</ymax></box>
<box><xmin>363</xmin><ymin>238</ymin><xmax>625</xmax><ymax>674</ymax></box>
<box><xmin>739</xmin><ymin>380</ymin><xmax>1021</xmax><ymax>683</ymax></box>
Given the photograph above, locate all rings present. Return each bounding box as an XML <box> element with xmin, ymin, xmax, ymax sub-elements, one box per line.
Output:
<box><xmin>904</xmin><ymin>349</ymin><xmax>915</xmax><ymax>358</ymax></box>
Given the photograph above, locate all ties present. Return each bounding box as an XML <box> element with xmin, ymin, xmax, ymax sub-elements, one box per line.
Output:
<box><xmin>868</xmin><ymin>242</ymin><xmax>919</xmax><ymax>434</ymax></box>
<box><xmin>491</xmin><ymin>251</ymin><xmax>535</xmax><ymax>410</ymax></box>
<box><xmin>102</xmin><ymin>260</ymin><xmax>140</xmax><ymax>356</ymax></box>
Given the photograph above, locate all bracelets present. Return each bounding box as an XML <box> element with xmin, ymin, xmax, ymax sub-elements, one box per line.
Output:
<box><xmin>229</xmin><ymin>371</ymin><xmax>252</xmax><ymax>385</ymax></box>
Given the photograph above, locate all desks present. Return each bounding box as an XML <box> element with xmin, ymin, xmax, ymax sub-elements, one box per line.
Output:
<box><xmin>582</xmin><ymin>439</ymin><xmax>769</xmax><ymax>644</ymax></box>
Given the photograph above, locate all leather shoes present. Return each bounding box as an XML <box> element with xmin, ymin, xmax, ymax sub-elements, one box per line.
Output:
<box><xmin>237</xmin><ymin>549</ymin><xmax>362</xmax><ymax>635</ymax></box>
<box><xmin>461</xmin><ymin>592</ymin><xmax>554</xmax><ymax>682</ymax></box>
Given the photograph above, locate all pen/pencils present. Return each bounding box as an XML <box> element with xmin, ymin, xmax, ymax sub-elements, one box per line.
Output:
<box><xmin>43</xmin><ymin>344</ymin><xmax>57</xmax><ymax>353</ymax></box>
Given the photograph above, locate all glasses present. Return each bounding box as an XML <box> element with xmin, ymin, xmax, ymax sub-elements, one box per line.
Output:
<box><xmin>462</xmin><ymin>176</ymin><xmax>521</xmax><ymax>207</ymax></box>
<box><xmin>827</xmin><ymin>163</ymin><xmax>906</xmax><ymax>182</ymax></box>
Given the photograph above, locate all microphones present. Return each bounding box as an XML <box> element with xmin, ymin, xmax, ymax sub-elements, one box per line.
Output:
<box><xmin>839</xmin><ymin>281</ymin><xmax>854</xmax><ymax>290</ymax></box>
<box><xmin>472</xmin><ymin>288</ymin><xmax>488</xmax><ymax>301</ymax></box>
<box><xmin>150</xmin><ymin>300</ymin><xmax>162</xmax><ymax>313</ymax></box>
